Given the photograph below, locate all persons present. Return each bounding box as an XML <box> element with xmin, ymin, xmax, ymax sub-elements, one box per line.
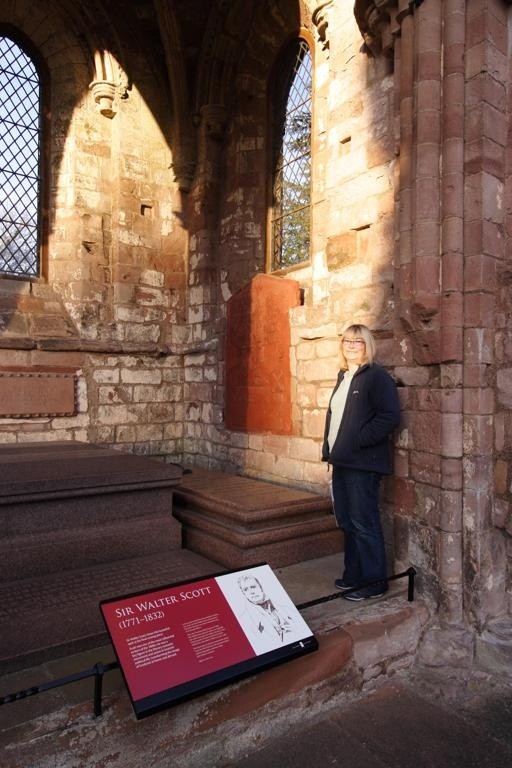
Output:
<box><xmin>236</xmin><ymin>571</ymin><xmax>295</xmax><ymax>648</ymax></box>
<box><xmin>319</xmin><ymin>321</ymin><xmax>402</xmax><ymax>603</ymax></box>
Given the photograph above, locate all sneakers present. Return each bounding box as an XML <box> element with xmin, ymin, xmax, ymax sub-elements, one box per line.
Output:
<box><xmin>335</xmin><ymin>579</ymin><xmax>384</xmax><ymax>601</ymax></box>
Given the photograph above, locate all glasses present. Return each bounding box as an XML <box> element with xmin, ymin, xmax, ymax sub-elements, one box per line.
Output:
<box><xmin>342</xmin><ymin>338</ymin><xmax>365</xmax><ymax>346</ymax></box>
